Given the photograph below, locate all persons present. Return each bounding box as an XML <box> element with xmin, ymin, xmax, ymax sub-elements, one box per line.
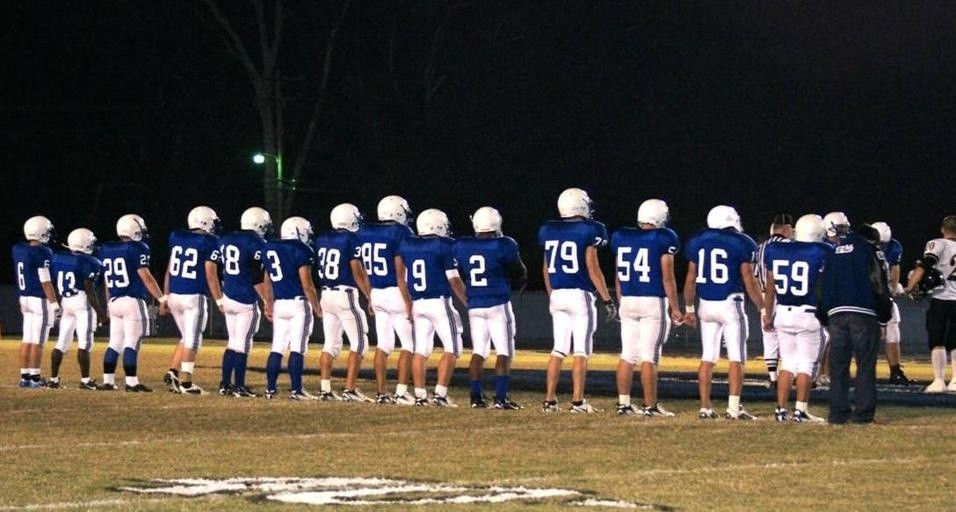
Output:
<box><xmin>537</xmin><ymin>187</ymin><xmax>617</xmax><ymax>412</ymax></box>
<box><xmin>611</xmin><ymin>199</ymin><xmax>683</xmax><ymax>418</ymax></box>
<box><xmin>683</xmin><ymin>205</ymin><xmax>766</xmax><ymax>421</ymax></box>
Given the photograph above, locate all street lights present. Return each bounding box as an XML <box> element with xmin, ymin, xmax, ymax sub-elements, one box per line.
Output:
<box><xmin>251</xmin><ymin>150</ymin><xmax>285</xmax><ymax>225</ymax></box>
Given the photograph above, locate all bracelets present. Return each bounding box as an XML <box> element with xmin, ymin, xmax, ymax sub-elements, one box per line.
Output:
<box><xmin>603</xmin><ymin>299</ymin><xmax>612</xmax><ymax>305</ymax></box>
<box><xmin>685</xmin><ymin>306</ymin><xmax>695</xmax><ymax>313</ymax></box>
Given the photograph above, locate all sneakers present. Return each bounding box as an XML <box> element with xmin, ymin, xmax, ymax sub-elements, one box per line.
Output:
<box><xmin>494</xmin><ymin>397</ymin><xmax>524</xmax><ymax>410</ymax></box>
<box><xmin>698</xmin><ymin>374</ymin><xmax>875</xmax><ymax>425</ymax></box>
<box><xmin>217</xmin><ymin>382</ymin><xmax>458</xmax><ymax>409</ymax></box>
<box><xmin>889</xmin><ymin>369</ymin><xmax>916</xmax><ymax>385</ymax></box>
<box><xmin>470</xmin><ymin>395</ymin><xmax>491</xmax><ymax>408</ymax></box>
<box><xmin>19</xmin><ymin>368</ymin><xmax>210</xmax><ymax>396</ymax></box>
<box><xmin>947</xmin><ymin>378</ymin><xmax>956</xmax><ymax>392</ymax></box>
<box><xmin>928</xmin><ymin>379</ymin><xmax>945</xmax><ymax>393</ymax></box>
<box><xmin>568</xmin><ymin>399</ymin><xmax>604</xmax><ymax>414</ymax></box>
<box><xmin>642</xmin><ymin>402</ymin><xmax>674</xmax><ymax>417</ymax></box>
<box><xmin>542</xmin><ymin>400</ymin><xmax>558</xmax><ymax>412</ymax></box>
<box><xmin>616</xmin><ymin>404</ymin><xmax>643</xmax><ymax>415</ymax></box>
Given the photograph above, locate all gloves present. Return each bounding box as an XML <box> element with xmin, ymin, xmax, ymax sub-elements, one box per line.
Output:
<box><xmin>604</xmin><ymin>299</ymin><xmax>616</xmax><ymax>323</ymax></box>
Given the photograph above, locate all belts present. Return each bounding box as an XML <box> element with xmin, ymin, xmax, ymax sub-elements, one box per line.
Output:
<box><xmin>787</xmin><ymin>307</ymin><xmax>817</xmax><ymax>312</ymax></box>
<box><xmin>276</xmin><ymin>297</ymin><xmax>305</xmax><ymax>300</ymax></box>
<box><xmin>321</xmin><ymin>287</ymin><xmax>352</xmax><ymax>292</ymax></box>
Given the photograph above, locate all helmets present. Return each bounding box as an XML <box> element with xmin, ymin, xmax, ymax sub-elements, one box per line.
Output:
<box><xmin>66</xmin><ymin>228</ymin><xmax>96</xmax><ymax>255</ymax></box>
<box><xmin>187</xmin><ymin>206</ymin><xmax>222</xmax><ymax>237</ymax></box>
<box><xmin>115</xmin><ymin>214</ymin><xmax>147</xmax><ymax>242</ymax></box>
<box><xmin>556</xmin><ymin>188</ymin><xmax>595</xmax><ymax>220</ymax></box>
<box><xmin>23</xmin><ymin>215</ymin><xmax>54</xmax><ymax>244</ymax></box>
<box><xmin>795</xmin><ymin>211</ymin><xmax>891</xmax><ymax>244</ymax></box>
<box><xmin>240</xmin><ymin>195</ymin><xmax>502</xmax><ymax>246</ymax></box>
<box><xmin>636</xmin><ymin>199</ymin><xmax>668</xmax><ymax>228</ymax></box>
<box><xmin>706</xmin><ymin>205</ymin><xmax>743</xmax><ymax>232</ymax></box>
<box><xmin>908</xmin><ymin>266</ymin><xmax>944</xmax><ymax>294</ymax></box>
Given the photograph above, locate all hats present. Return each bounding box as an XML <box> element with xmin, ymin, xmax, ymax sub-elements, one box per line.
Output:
<box><xmin>774</xmin><ymin>213</ymin><xmax>793</xmax><ymax>226</ymax></box>
